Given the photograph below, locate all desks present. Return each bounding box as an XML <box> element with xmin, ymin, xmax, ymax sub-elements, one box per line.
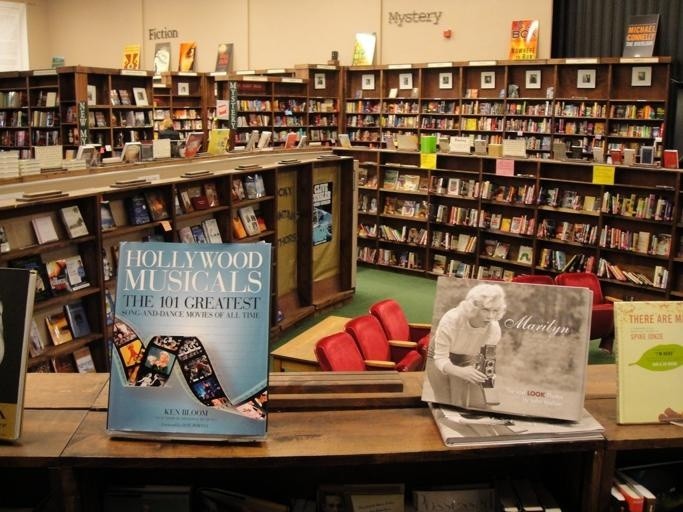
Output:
<box><xmin>270</xmin><ymin>315</ymin><xmax>353</xmax><ymax>373</ymax></box>
<box><xmin>2</xmin><ymin>303</ymin><xmax>649</xmax><ymax>512</ymax></box>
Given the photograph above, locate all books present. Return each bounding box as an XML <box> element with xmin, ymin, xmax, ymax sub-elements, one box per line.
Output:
<box><xmin>421</xmin><ymin>276</ymin><xmax>605</xmax><ymax>448</ymax></box>
<box><xmin>509</xmin><ymin>18</ymin><xmax>539</xmax><ymax>61</ymax></box>
<box><xmin>357</xmin><ymin>161</ymin><xmax>672</xmax><ymax>290</ymax></box>
<box><xmin>64</xmin><ymin>85</ymin><xmax>203</xmax><ymax>162</ymax></box>
<box><xmin>342</xmin><ymin>86</ymin><xmax>665</xmax><ymax>161</ymax></box>
<box><xmin>51</xmin><ymin>56</ymin><xmax>65</xmax><ymax>69</ymax></box>
<box><xmin>121</xmin><ymin>44</ymin><xmax>140</xmax><ymax>71</ymax></box>
<box><xmin>351</xmin><ymin>30</ymin><xmax>377</xmax><ymax>65</ymax></box>
<box><xmin>217</xmin><ymin>43</ymin><xmax>233</xmax><ymax>73</ymax></box>
<box><xmin>357</xmin><ymin>169</ymin><xmax>671</xmax><ymax>289</ymax></box>
<box><xmin>113</xmin><ymin>240</ymin><xmax>268</xmax><ymax>441</ymax></box>
<box><xmin>208</xmin><ymin>98</ymin><xmax>338</xmax><ymax>154</ymax></box>
<box><xmin>1</xmin><ymin>178</ymin><xmax>307</xmax><ymax>371</ymax></box>
<box><xmin>0</xmin><ymin>90</ymin><xmax>59</xmax><ymax>170</ymax></box>
<box><xmin>0</xmin><ymin>268</ymin><xmax>36</xmax><ymax>442</ymax></box>
<box><xmin>123</xmin><ymin>472</ymin><xmax>657</xmax><ymax>512</ymax></box>
<box><xmin>612</xmin><ymin>302</ymin><xmax>683</xmax><ymax>424</ymax></box>
<box><xmin>151</xmin><ymin>40</ymin><xmax>198</xmax><ymax>74</ymax></box>
<box><xmin>621</xmin><ymin>12</ymin><xmax>660</xmax><ymax>57</ymax></box>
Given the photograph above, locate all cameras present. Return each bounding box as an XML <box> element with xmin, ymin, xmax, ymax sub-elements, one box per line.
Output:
<box><xmin>476</xmin><ymin>345</ymin><xmax>501</xmax><ymax>405</ymax></box>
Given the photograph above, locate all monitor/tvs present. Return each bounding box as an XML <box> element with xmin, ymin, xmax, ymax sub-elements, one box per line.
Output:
<box><xmin>158</xmin><ymin>131</ymin><xmax>180</xmax><ymax>140</ymax></box>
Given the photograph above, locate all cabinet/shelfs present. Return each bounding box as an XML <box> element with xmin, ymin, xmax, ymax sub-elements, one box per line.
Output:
<box><xmin>323</xmin><ymin>145</ymin><xmax>682</xmax><ymax>298</ymax></box>
<box><xmin>2</xmin><ymin>67</ymin><xmax>63</xmax><ymax>147</ymax></box>
<box><xmin>54</xmin><ymin>61</ymin><xmax>342</xmax><ymax>150</ymax></box>
<box><xmin>0</xmin><ymin>151</ymin><xmax>353</xmax><ymax>374</ymax></box>
<box><xmin>343</xmin><ymin>56</ymin><xmax>675</xmax><ymax>168</ymax></box>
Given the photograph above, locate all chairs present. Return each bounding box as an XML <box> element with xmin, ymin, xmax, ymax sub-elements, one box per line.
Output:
<box><xmin>314</xmin><ymin>299</ymin><xmax>431</xmax><ymax>372</ymax></box>
<box><xmin>480</xmin><ymin>274</ymin><xmax>615</xmax><ymax>355</ymax></box>
